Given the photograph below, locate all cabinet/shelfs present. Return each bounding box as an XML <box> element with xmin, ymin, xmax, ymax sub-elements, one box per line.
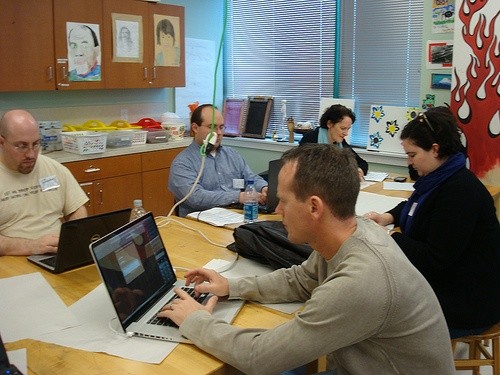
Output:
<box><xmin>61</xmin><ymin>146</ymin><xmax>186</xmax><ymax>223</ymax></box>
<box><xmin>104</xmin><ymin>0</ymin><xmax>186</xmax><ymax>89</ymax></box>
<box><xmin>0</xmin><ymin>0</ymin><xmax>105</xmax><ymax>91</ymax></box>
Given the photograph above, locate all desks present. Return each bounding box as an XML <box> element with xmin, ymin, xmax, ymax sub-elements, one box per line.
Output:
<box><xmin>0</xmin><ymin>172</ymin><xmax>500</xmax><ymax>375</ymax></box>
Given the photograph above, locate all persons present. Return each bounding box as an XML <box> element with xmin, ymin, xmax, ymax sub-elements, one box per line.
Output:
<box><xmin>113</xmin><ymin>288</ymin><xmax>143</xmax><ymax>320</ymax></box>
<box><xmin>362</xmin><ymin>106</ymin><xmax>500</xmax><ymax>339</ymax></box>
<box><xmin>168</xmin><ymin>104</ymin><xmax>268</xmax><ymax>217</ymax></box>
<box><xmin>155</xmin><ymin>143</ymin><xmax>455</xmax><ymax>375</ymax></box>
<box><xmin>0</xmin><ymin>108</ymin><xmax>90</xmax><ymax>256</ymax></box>
<box><xmin>298</xmin><ymin>104</ymin><xmax>368</xmax><ymax>182</ymax></box>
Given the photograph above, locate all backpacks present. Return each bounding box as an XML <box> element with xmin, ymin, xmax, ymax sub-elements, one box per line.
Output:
<box><xmin>227</xmin><ymin>221</ymin><xmax>314</xmax><ymax>271</ymax></box>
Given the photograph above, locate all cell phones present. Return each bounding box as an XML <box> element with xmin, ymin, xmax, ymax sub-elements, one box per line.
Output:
<box><xmin>394</xmin><ymin>177</ymin><xmax>407</xmax><ymax>182</ymax></box>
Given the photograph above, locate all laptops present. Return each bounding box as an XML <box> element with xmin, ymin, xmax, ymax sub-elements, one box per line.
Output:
<box><xmin>89</xmin><ymin>210</ymin><xmax>247</xmax><ymax>345</ymax></box>
<box><xmin>26</xmin><ymin>208</ymin><xmax>133</xmax><ymax>274</ymax></box>
<box><xmin>232</xmin><ymin>158</ymin><xmax>282</xmax><ymax>213</ymax></box>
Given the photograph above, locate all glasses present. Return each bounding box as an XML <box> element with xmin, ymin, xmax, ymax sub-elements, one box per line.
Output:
<box><xmin>200</xmin><ymin>124</ymin><xmax>228</xmax><ymax>131</ymax></box>
<box><xmin>1</xmin><ymin>135</ymin><xmax>42</xmax><ymax>151</ymax></box>
<box><xmin>417</xmin><ymin>112</ymin><xmax>435</xmax><ymax>132</ymax></box>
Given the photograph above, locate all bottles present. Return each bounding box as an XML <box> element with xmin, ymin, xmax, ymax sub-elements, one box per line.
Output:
<box><xmin>129</xmin><ymin>199</ymin><xmax>147</xmax><ymax>222</ymax></box>
<box><xmin>243</xmin><ymin>180</ymin><xmax>259</xmax><ymax>224</ymax></box>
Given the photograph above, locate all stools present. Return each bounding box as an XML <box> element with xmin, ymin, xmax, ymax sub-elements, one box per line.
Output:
<box><xmin>451</xmin><ymin>324</ymin><xmax>500</xmax><ymax>375</ymax></box>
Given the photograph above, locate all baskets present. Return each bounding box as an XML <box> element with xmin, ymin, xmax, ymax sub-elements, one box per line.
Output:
<box><xmin>58</xmin><ymin>130</ymin><xmax>108</xmax><ymax>155</ymax></box>
<box><xmin>106</xmin><ymin>129</ymin><xmax>170</xmax><ymax>148</ymax></box>
